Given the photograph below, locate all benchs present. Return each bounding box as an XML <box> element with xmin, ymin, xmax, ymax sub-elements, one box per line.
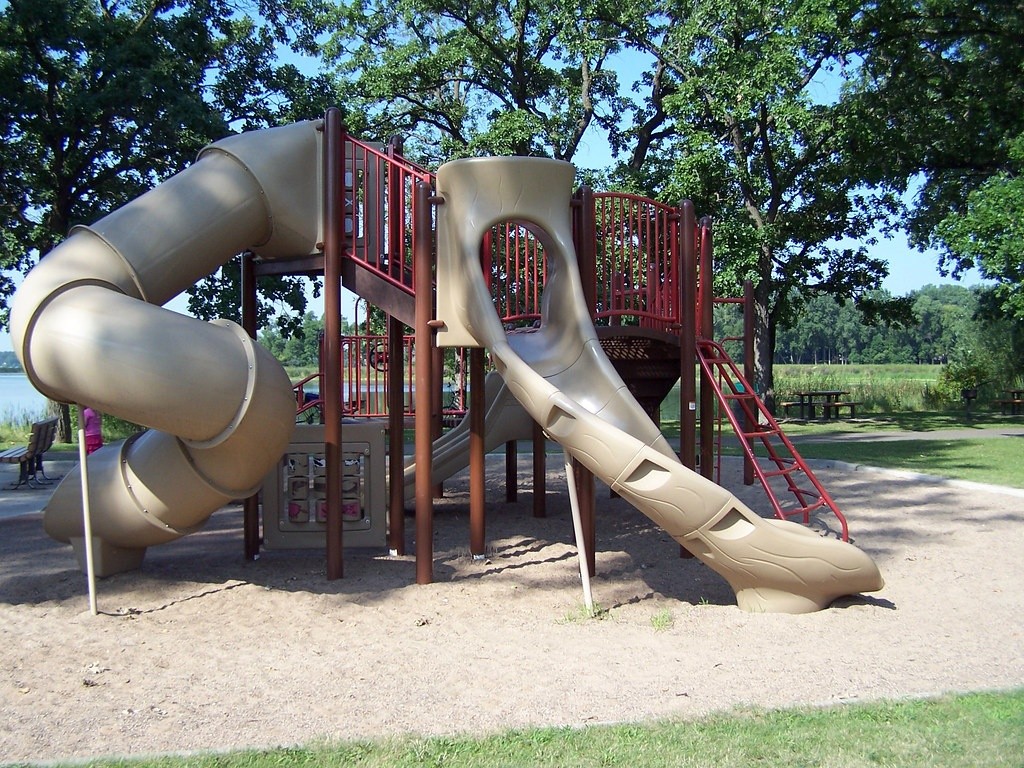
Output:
<box><xmin>820</xmin><ymin>402</ymin><xmax>864</xmax><ymax>421</ymax></box>
<box><xmin>0</xmin><ymin>415</ymin><xmax>61</xmax><ymax>492</ymax></box>
<box><xmin>996</xmin><ymin>399</ymin><xmax>1024</xmax><ymax>416</ymax></box>
<box><xmin>780</xmin><ymin>402</ymin><xmax>824</xmax><ymax>421</ymax></box>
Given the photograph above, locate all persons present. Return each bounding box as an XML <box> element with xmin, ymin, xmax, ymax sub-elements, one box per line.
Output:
<box><xmin>84</xmin><ymin>406</ymin><xmax>103</xmax><ymax>454</ymax></box>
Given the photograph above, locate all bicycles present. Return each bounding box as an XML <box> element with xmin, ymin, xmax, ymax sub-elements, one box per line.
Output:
<box><xmin>443</xmin><ymin>382</ymin><xmax>469</xmax><ymax>418</ymax></box>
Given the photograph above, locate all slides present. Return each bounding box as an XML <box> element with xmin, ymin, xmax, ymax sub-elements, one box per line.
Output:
<box><xmin>7</xmin><ymin>119</ymin><xmax>323</xmax><ymax>543</ymax></box>
<box><xmin>310</xmin><ymin>407</ymin><xmax>495</xmax><ymax>514</ymax></box>
<box><xmin>443</xmin><ymin>330</ymin><xmax>885</xmax><ymax>614</ymax></box>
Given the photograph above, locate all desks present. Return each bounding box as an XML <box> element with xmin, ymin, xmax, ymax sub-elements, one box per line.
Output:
<box><xmin>788</xmin><ymin>391</ymin><xmax>850</xmax><ymax>421</ymax></box>
<box><xmin>1000</xmin><ymin>389</ymin><xmax>1024</xmax><ymax>415</ymax></box>
